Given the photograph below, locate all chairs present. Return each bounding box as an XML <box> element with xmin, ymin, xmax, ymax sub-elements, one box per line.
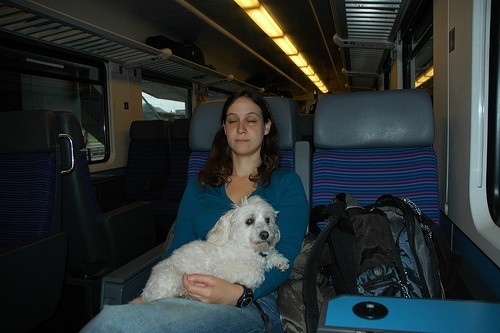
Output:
<box><xmin>0</xmin><ymin>88</ymin><xmax>464</xmax><ymax>333</ymax></box>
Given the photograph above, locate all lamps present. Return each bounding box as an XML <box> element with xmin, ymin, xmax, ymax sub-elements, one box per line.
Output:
<box><xmin>234</xmin><ymin>0</ymin><xmax>328</xmax><ymax>93</ymax></box>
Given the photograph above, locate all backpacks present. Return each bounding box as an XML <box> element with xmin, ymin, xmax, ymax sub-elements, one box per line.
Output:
<box><xmin>275</xmin><ymin>192</ymin><xmax>445</xmax><ymax>333</ymax></box>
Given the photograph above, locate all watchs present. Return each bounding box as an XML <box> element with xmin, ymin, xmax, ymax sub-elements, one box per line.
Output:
<box><xmin>234</xmin><ymin>282</ymin><xmax>253</xmax><ymax>308</ymax></box>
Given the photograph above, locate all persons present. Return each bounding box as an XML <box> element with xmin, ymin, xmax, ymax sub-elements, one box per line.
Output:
<box><xmin>79</xmin><ymin>91</ymin><xmax>308</xmax><ymax>333</ymax></box>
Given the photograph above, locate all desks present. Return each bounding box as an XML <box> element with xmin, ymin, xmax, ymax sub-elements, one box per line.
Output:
<box><xmin>315</xmin><ymin>293</ymin><xmax>500</xmax><ymax>333</ymax></box>
<box><xmin>90</xmin><ymin>174</ymin><xmax>118</xmax><ymax>201</ymax></box>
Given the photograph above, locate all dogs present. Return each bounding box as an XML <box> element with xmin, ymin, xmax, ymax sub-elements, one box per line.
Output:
<box><xmin>142</xmin><ymin>195</ymin><xmax>290</xmax><ymax>303</ymax></box>
